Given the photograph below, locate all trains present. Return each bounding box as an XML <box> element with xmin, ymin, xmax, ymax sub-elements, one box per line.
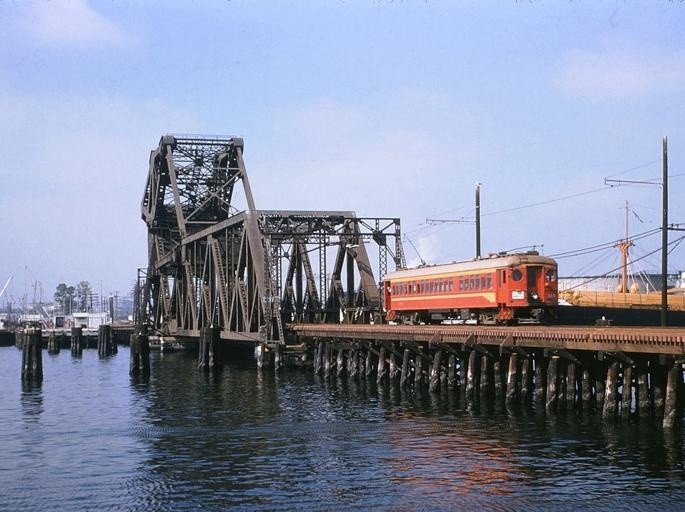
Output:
<box><xmin>382</xmin><ymin>251</ymin><xmax>558</xmax><ymax>326</ymax></box>
